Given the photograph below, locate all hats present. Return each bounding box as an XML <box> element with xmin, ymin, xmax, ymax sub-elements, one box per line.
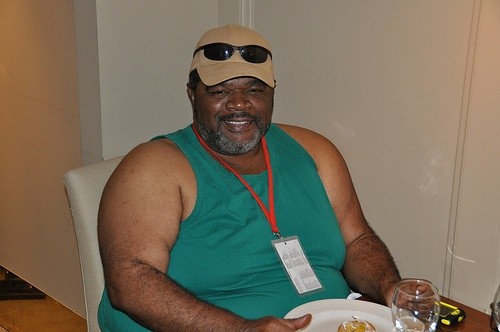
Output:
<box><xmin>189</xmin><ymin>24</ymin><xmax>274</xmax><ymax>88</ymax></box>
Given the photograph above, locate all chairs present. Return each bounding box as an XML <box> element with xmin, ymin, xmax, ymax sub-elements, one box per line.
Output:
<box><xmin>64</xmin><ymin>154</ymin><xmax>126</xmax><ymax>332</ymax></box>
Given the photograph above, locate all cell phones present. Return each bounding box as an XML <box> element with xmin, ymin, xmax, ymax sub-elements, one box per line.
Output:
<box><xmin>433</xmin><ymin>301</ymin><xmax>466</xmax><ymax>324</ymax></box>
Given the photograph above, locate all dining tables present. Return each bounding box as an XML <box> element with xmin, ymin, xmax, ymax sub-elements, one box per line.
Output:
<box><xmin>356</xmin><ymin>294</ymin><xmax>499</xmax><ymax>332</ymax></box>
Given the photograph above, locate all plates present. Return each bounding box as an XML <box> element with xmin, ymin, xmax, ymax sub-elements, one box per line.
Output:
<box><xmin>283</xmin><ymin>299</ymin><xmax>400</xmax><ymax>332</ymax></box>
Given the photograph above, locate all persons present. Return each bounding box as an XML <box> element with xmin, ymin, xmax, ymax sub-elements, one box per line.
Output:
<box><xmin>97</xmin><ymin>23</ymin><xmax>435</xmax><ymax>332</ymax></box>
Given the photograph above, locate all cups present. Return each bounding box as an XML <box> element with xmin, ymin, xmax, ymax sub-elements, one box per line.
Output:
<box><xmin>391</xmin><ymin>278</ymin><xmax>440</xmax><ymax>332</ymax></box>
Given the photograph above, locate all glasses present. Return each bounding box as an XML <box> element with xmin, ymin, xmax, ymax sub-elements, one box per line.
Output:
<box><xmin>192</xmin><ymin>42</ymin><xmax>273</xmax><ymax>64</ymax></box>
